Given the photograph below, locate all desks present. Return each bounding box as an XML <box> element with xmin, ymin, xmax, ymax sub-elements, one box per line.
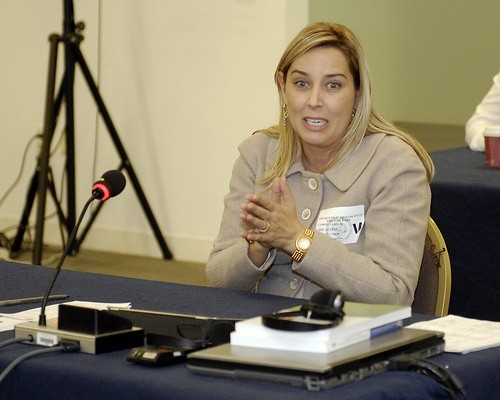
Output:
<box><xmin>0</xmin><ymin>261</ymin><xmax>500</xmax><ymax>400</ymax></box>
<box><xmin>427</xmin><ymin>145</ymin><xmax>500</xmax><ymax>321</ymax></box>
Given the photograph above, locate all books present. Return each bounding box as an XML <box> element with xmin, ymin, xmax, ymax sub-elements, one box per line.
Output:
<box><xmin>229</xmin><ymin>301</ymin><xmax>413</xmax><ymax>354</ymax></box>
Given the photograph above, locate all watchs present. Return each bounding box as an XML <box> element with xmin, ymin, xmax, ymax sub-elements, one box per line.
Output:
<box><xmin>291</xmin><ymin>228</ymin><xmax>314</xmax><ymax>262</ymax></box>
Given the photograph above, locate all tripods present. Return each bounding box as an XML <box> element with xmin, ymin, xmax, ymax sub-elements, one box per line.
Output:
<box><xmin>9</xmin><ymin>0</ymin><xmax>174</xmax><ymax>263</ymax></box>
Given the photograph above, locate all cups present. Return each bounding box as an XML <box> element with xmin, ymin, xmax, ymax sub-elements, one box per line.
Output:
<box><xmin>483</xmin><ymin>125</ymin><xmax>500</xmax><ymax>168</ymax></box>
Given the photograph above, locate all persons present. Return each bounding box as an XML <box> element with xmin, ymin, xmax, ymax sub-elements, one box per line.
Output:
<box><xmin>465</xmin><ymin>73</ymin><xmax>500</xmax><ymax>150</ymax></box>
<box><xmin>206</xmin><ymin>23</ymin><xmax>434</xmax><ymax>305</ymax></box>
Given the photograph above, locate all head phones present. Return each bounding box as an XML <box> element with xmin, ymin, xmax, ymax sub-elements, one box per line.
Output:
<box><xmin>261</xmin><ymin>288</ymin><xmax>345</xmax><ymax>332</ymax></box>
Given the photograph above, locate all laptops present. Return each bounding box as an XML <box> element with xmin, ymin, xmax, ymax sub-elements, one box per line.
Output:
<box><xmin>186</xmin><ymin>327</ymin><xmax>446</xmax><ymax>391</ymax></box>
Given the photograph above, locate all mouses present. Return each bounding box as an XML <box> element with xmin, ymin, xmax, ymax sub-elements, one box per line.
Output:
<box><xmin>171</xmin><ymin>319</ymin><xmax>235</xmax><ymax>351</ymax></box>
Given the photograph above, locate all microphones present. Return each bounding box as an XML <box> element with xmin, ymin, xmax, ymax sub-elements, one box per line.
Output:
<box><xmin>15</xmin><ymin>169</ymin><xmax>146</xmax><ymax>354</ymax></box>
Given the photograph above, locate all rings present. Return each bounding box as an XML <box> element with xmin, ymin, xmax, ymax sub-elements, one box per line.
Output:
<box><xmin>259</xmin><ymin>219</ymin><xmax>270</xmax><ymax>232</ymax></box>
